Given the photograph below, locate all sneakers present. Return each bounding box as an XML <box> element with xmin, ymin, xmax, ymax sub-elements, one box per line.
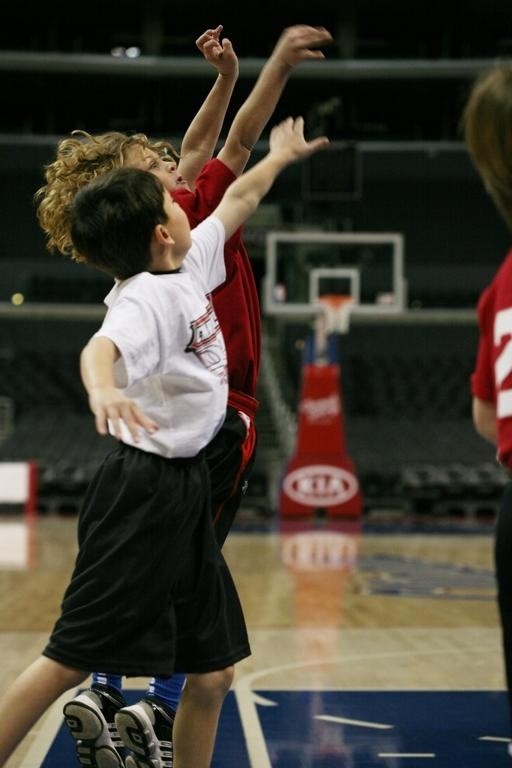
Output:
<box><xmin>64</xmin><ymin>683</ymin><xmax>125</xmax><ymax>768</ymax></box>
<box><xmin>114</xmin><ymin>694</ymin><xmax>173</xmax><ymax>768</ymax></box>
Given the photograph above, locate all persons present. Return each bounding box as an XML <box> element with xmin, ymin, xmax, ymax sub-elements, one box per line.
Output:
<box><xmin>34</xmin><ymin>20</ymin><xmax>331</xmax><ymax>766</ymax></box>
<box><xmin>456</xmin><ymin>65</ymin><xmax>512</xmax><ymax>717</ymax></box>
<box><xmin>0</xmin><ymin>112</ymin><xmax>331</xmax><ymax>767</ymax></box>
